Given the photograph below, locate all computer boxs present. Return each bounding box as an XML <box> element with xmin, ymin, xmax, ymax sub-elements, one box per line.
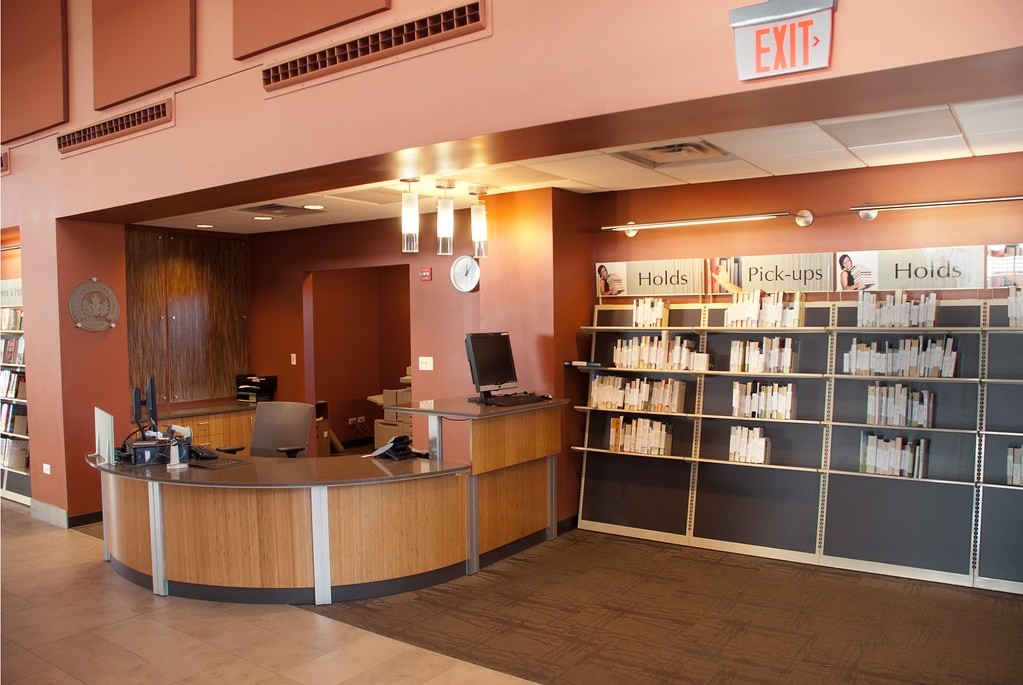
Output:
<box><xmin>125</xmin><ymin>441</ymin><xmax>189</xmax><ymax>465</ymax></box>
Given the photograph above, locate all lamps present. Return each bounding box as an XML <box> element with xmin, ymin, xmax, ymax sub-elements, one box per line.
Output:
<box><xmin>436</xmin><ymin>178</ymin><xmax>454</xmax><ymax>255</ymax></box>
<box><xmin>600</xmin><ymin>210</ymin><xmax>813</xmax><ymax>237</ymax></box>
<box><xmin>850</xmin><ymin>196</ymin><xmax>1023</xmax><ymax>221</ymax></box>
<box><xmin>468</xmin><ymin>186</ymin><xmax>488</xmax><ymax>258</ymax></box>
<box><xmin>400</xmin><ymin>176</ymin><xmax>419</xmax><ymax>253</ymax></box>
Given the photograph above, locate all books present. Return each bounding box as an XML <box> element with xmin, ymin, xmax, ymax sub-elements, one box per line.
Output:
<box><xmin>703</xmin><ymin>257</ymin><xmax>742</xmax><ymax>293</ymax></box>
<box><xmin>613</xmin><ymin>334</ymin><xmax>710</xmax><ymax>371</ymax></box>
<box><xmin>859</xmin><ymin>430</ymin><xmax>930</xmax><ymax>479</ymax></box>
<box><xmin>730</xmin><ymin>337</ymin><xmax>800</xmax><ymax>373</ymax></box>
<box><xmin>606</xmin><ymin>273</ymin><xmax>625</xmax><ymax>295</ymax></box>
<box><xmin>724</xmin><ymin>289</ymin><xmax>807</xmax><ymax>328</ymax></box>
<box><xmin>729</xmin><ymin>426</ymin><xmax>771</xmax><ymax>465</ymax></box>
<box><xmin>844</xmin><ymin>335</ymin><xmax>957</xmax><ymax>378</ymax></box>
<box><xmin>632</xmin><ymin>297</ymin><xmax>670</xmax><ymax>327</ymax></box>
<box><xmin>609</xmin><ymin>416</ymin><xmax>674</xmax><ymax>455</ymax></box>
<box><xmin>867</xmin><ymin>380</ymin><xmax>935</xmax><ymax>428</ymax></box>
<box><xmin>1</xmin><ymin>306</ymin><xmax>30</xmax><ymax>471</ymax></box>
<box><xmin>732</xmin><ymin>379</ymin><xmax>799</xmax><ymax>420</ymax></box>
<box><xmin>849</xmin><ymin>264</ymin><xmax>875</xmax><ymax>290</ymax></box>
<box><xmin>857</xmin><ymin>290</ymin><xmax>939</xmax><ymax>327</ymax></box>
<box><xmin>1006</xmin><ymin>444</ymin><xmax>1023</xmax><ymax>487</ymax></box>
<box><xmin>1008</xmin><ymin>286</ymin><xmax>1023</xmax><ymax>327</ymax></box>
<box><xmin>591</xmin><ymin>375</ymin><xmax>686</xmax><ymax>413</ymax></box>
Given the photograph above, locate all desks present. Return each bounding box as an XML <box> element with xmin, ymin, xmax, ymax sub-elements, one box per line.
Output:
<box><xmin>82</xmin><ymin>453</ymin><xmax>472</xmax><ymax>605</ymax></box>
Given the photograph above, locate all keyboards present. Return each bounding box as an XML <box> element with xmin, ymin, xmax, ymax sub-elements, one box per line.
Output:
<box><xmin>190</xmin><ymin>445</ymin><xmax>219</xmax><ymax>461</ymax></box>
<box><xmin>488</xmin><ymin>393</ymin><xmax>544</xmax><ymax>407</ymax></box>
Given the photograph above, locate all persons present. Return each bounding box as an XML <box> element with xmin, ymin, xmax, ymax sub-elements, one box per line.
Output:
<box><xmin>839</xmin><ymin>255</ymin><xmax>864</xmax><ymax>290</ymax></box>
<box><xmin>598</xmin><ymin>265</ymin><xmax>620</xmax><ymax>295</ymax></box>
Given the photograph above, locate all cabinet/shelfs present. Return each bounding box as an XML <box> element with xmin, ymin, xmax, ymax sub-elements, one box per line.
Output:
<box><xmin>0</xmin><ymin>305</ymin><xmax>30</xmax><ymax>477</ymax></box>
<box><xmin>125</xmin><ymin>223</ymin><xmax>256</xmax><ymax>457</ymax></box>
<box><xmin>315</xmin><ymin>419</ymin><xmax>330</xmax><ymax>456</ymax></box>
<box><xmin>571</xmin><ymin>295</ymin><xmax>1023</xmax><ymax>593</ymax></box>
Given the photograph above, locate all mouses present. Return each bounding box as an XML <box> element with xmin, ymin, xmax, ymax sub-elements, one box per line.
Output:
<box><xmin>539</xmin><ymin>393</ymin><xmax>553</xmax><ymax>400</ymax></box>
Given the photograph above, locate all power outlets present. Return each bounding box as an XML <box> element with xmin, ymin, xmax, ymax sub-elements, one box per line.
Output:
<box><xmin>349</xmin><ymin>417</ymin><xmax>367</xmax><ymax>425</ymax></box>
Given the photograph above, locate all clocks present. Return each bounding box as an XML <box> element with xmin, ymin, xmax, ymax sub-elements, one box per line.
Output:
<box><xmin>449</xmin><ymin>255</ymin><xmax>480</xmax><ymax>292</ymax></box>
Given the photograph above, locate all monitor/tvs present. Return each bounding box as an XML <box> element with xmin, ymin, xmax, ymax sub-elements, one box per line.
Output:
<box><xmin>465</xmin><ymin>332</ymin><xmax>519</xmax><ymax>402</ymax></box>
<box><xmin>132</xmin><ymin>376</ymin><xmax>157</xmax><ymax>445</ymax></box>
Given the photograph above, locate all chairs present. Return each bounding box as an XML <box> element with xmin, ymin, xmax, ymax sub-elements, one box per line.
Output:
<box><xmin>216</xmin><ymin>400</ymin><xmax>314</xmax><ymax>457</ymax></box>
